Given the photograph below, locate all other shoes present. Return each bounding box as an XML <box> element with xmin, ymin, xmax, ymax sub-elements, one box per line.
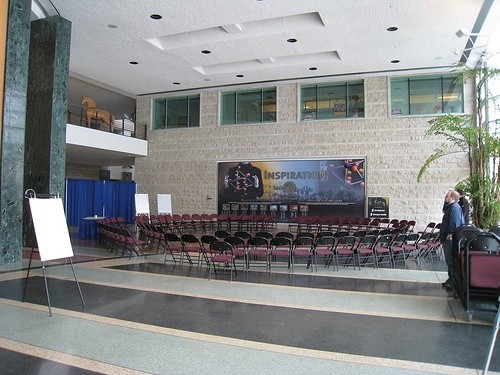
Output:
<box><xmin>442</xmin><ymin>279</ymin><xmax>452</xmax><ymax>290</ymax></box>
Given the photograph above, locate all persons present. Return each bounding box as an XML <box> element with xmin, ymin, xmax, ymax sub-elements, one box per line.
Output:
<box><xmin>439</xmin><ymin>189</ymin><xmax>466</xmax><ymax>289</ymax></box>
<box><xmin>220</xmin><ymin>162</ymin><xmax>264</xmax><ymax>202</ymax></box>
<box><xmin>456</xmin><ymin>189</ymin><xmax>470</xmax><ymax>227</ymax></box>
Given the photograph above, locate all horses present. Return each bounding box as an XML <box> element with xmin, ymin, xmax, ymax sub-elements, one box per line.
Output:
<box><xmin>81</xmin><ymin>95</ymin><xmax>115</xmax><ymax>132</ymax></box>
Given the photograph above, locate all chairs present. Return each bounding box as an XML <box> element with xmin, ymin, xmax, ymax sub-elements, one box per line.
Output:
<box><xmin>97</xmin><ymin>214</ymin><xmax>442</xmax><ymax>273</ymax></box>
<box><xmin>207</xmin><ymin>241</ymin><xmax>237</xmax><ymax>282</ymax></box>
<box><xmin>453</xmin><ymin>223</ymin><xmax>500</xmax><ymax>321</ymax></box>
